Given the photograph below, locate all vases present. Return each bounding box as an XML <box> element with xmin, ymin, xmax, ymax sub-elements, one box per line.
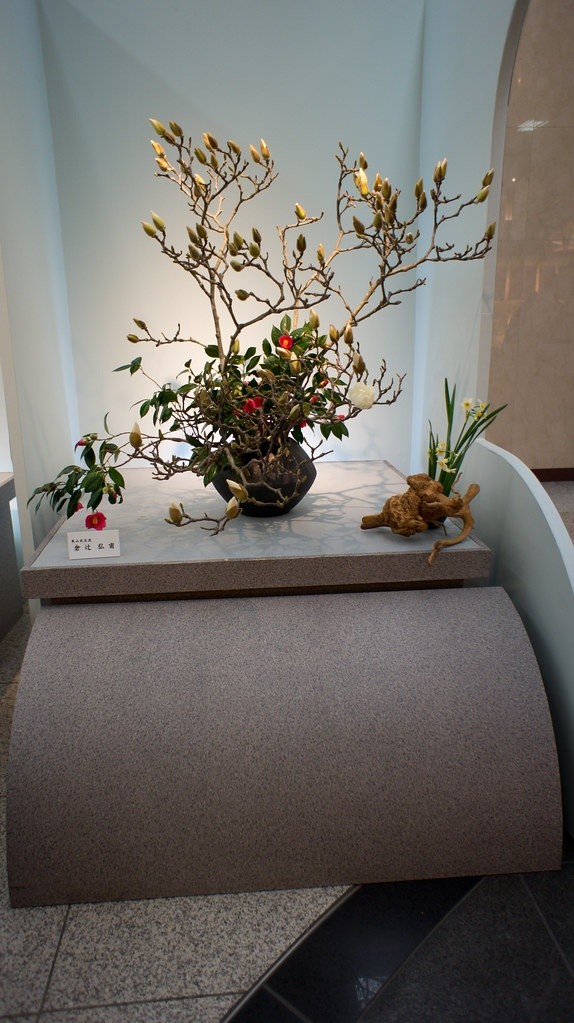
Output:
<box><xmin>211</xmin><ymin>435</ymin><xmax>318</xmax><ymax>517</ymax></box>
<box><xmin>427</xmin><ymin>516</ymin><xmax>446</xmax><ymax>529</ymax></box>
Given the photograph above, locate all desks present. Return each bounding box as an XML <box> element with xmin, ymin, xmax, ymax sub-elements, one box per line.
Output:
<box><xmin>16</xmin><ymin>459</ymin><xmax>492</xmax><ymax>608</ymax></box>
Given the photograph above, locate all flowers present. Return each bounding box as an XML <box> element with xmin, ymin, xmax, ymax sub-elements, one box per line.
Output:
<box><xmin>22</xmin><ymin>119</ymin><xmax>496</xmax><ymax>533</ymax></box>
<box><xmin>427</xmin><ymin>375</ymin><xmax>514</xmax><ymax>496</ymax></box>
